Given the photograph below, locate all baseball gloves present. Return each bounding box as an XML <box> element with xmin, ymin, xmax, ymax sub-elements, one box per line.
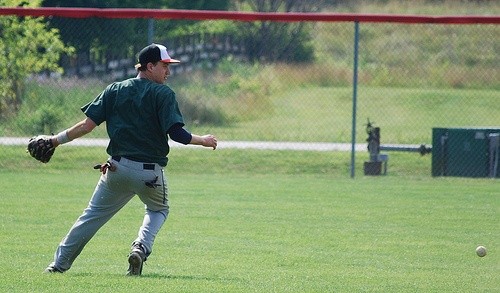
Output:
<box><xmin>27</xmin><ymin>133</ymin><xmax>57</xmax><ymax>164</ymax></box>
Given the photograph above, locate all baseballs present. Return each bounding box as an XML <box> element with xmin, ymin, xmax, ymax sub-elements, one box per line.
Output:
<box><xmin>476</xmin><ymin>245</ymin><xmax>487</xmax><ymax>257</ymax></box>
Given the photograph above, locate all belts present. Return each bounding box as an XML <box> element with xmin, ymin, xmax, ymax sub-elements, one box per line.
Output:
<box><xmin>112</xmin><ymin>155</ymin><xmax>155</xmax><ymax>170</ymax></box>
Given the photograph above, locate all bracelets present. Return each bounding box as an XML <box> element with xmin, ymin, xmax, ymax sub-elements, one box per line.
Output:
<box><xmin>56</xmin><ymin>130</ymin><xmax>71</xmax><ymax>144</ymax></box>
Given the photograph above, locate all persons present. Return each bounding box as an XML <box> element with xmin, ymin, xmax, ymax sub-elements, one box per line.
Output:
<box><xmin>26</xmin><ymin>43</ymin><xmax>217</xmax><ymax>277</ymax></box>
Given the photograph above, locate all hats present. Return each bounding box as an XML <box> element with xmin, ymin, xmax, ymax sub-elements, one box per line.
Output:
<box><xmin>137</xmin><ymin>43</ymin><xmax>181</xmax><ymax>64</ymax></box>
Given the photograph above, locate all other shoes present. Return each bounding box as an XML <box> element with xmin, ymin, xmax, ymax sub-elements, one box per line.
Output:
<box><xmin>127</xmin><ymin>249</ymin><xmax>143</xmax><ymax>276</ymax></box>
<box><xmin>42</xmin><ymin>262</ymin><xmax>60</xmax><ymax>273</ymax></box>
